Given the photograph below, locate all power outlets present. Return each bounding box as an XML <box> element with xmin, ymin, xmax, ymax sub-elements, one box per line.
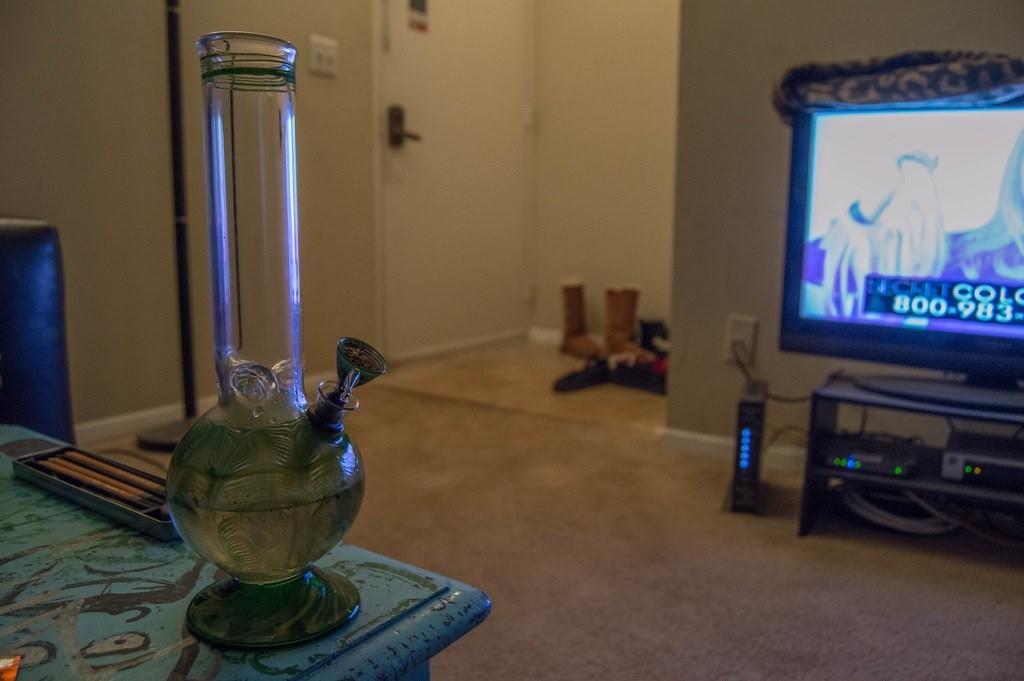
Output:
<box><xmin>724</xmin><ymin>315</ymin><xmax>760</xmax><ymax>368</ymax></box>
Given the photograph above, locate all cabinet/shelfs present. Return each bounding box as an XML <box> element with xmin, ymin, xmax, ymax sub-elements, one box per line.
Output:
<box><xmin>795</xmin><ymin>372</ymin><xmax>1024</xmax><ymax>538</ymax></box>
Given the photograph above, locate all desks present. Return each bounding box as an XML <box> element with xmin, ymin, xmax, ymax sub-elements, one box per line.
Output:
<box><xmin>0</xmin><ymin>424</ymin><xmax>493</xmax><ymax>681</ymax></box>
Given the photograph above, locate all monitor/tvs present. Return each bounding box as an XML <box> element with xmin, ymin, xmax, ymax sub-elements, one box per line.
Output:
<box><xmin>778</xmin><ymin>110</ymin><xmax>1024</xmax><ymax>413</ymax></box>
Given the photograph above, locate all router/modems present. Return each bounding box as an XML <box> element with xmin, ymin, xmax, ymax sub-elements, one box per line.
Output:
<box><xmin>825</xmin><ymin>436</ymin><xmax>919</xmax><ymax>478</ymax></box>
